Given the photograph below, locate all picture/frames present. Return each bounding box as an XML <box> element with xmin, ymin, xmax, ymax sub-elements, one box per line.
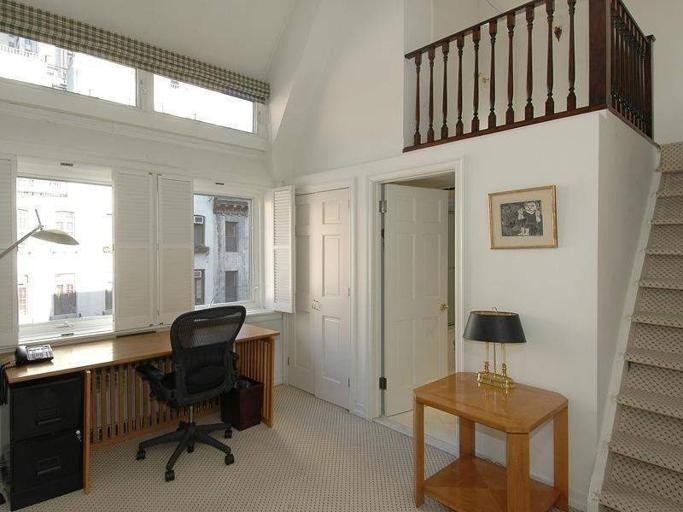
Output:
<box><xmin>488</xmin><ymin>185</ymin><xmax>558</xmax><ymax>249</ymax></box>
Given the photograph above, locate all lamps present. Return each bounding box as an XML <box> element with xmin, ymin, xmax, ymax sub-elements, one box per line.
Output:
<box><xmin>0</xmin><ymin>207</ymin><xmax>79</xmax><ymax>257</ymax></box>
<box><xmin>462</xmin><ymin>308</ymin><xmax>526</xmax><ymax>388</ymax></box>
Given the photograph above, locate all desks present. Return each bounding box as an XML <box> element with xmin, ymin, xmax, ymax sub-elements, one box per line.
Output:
<box><xmin>411</xmin><ymin>373</ymin><xmax>568</xmax><ymax>512</ymax></box>
<box><xmin>0</xmin><ymin>322</ymin><xmax>279</xmax><ymax>512</ymax></box>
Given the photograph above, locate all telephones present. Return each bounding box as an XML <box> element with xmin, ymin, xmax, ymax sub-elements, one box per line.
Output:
<box><xmin>14</xmin><ymin>344</ymin><xmax>55</xmax><ymax>366</ymax></box>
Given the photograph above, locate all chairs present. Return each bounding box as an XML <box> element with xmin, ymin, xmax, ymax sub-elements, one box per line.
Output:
<box><xmin>134</xmin><ymin>306</ymin><xmax>245</xmax><ymax>481</ymax></box>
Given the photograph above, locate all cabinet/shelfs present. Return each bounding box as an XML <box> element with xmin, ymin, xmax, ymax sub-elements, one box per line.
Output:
<box><xmin>0</xmin><ymin>368</ymin><xmax>84</xmax><ymax>512</ymax></box>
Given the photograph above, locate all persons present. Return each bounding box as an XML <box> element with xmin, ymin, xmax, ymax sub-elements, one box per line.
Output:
<box><xmin>511</xmin><ymin>201</ymin><xmax>542</xmax><ymax>236</ymax></box>
